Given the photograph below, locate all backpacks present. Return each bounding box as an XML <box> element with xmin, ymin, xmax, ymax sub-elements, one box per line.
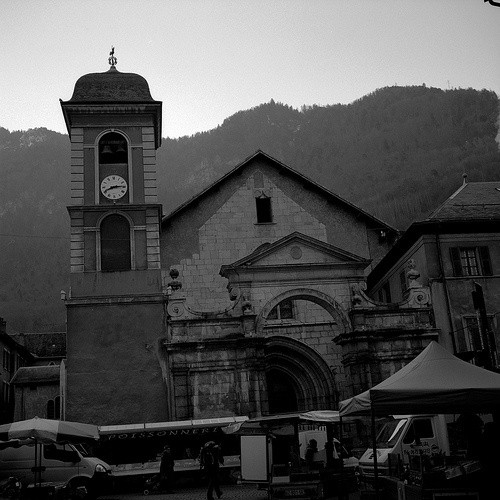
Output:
<box><xmin>199</xmin><ymin>447</ymin><xmax>213</xmax><ymax>466</ymax></box>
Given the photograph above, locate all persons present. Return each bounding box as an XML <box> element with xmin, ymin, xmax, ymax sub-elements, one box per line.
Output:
<box><xmin>305</xmin><ymin>444</ymin><xmax>315</xmax><ymax>473</ymax></box>
<box><xmin>159</xmin><ymin>445</ymin><xmax>175</xmax><ymax>493</ymax></box>
<box><xmin>198</xmin><ymin>435</ymin><xmax>224</xmax><ymax>500</ymax></box>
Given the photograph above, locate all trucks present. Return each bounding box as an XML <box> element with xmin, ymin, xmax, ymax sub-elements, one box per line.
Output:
<box><xmin>356</xmin><ymin>413</ymin><xmax>494</xmax><ymax>491</ymax></box>
<box><xmin>0</xmin><ymin>436</ymin><xmax>113</xmax><ymax>499</ymax></box>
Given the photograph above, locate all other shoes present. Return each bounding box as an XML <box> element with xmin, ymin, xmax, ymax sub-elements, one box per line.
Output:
<box><xmin>217</xmin><ymin>491</ymin><xmax>222</xmax><ymax>497</ymax></box>
<box><xmin>207</xmin><ymin>497</ymin><xmax>214</xmax><ymax>500</ymax></box>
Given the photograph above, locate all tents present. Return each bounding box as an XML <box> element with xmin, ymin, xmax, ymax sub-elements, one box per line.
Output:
<box><xmin>339</xmin><ymin>341</ymin><xmax>500</xmax><ymax>492</ymax></box>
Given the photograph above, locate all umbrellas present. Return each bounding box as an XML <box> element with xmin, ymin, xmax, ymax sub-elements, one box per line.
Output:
<box><xmin>0</xmin><ymin>416</ymin><xmax>99</xmax><ymax>489</ymax></box>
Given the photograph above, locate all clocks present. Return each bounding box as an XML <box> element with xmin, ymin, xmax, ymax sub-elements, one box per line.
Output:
<box><xmin>100</xmin><ymin>174</ymin><xmax>128</xmax><ymax>200</ymax></box>
